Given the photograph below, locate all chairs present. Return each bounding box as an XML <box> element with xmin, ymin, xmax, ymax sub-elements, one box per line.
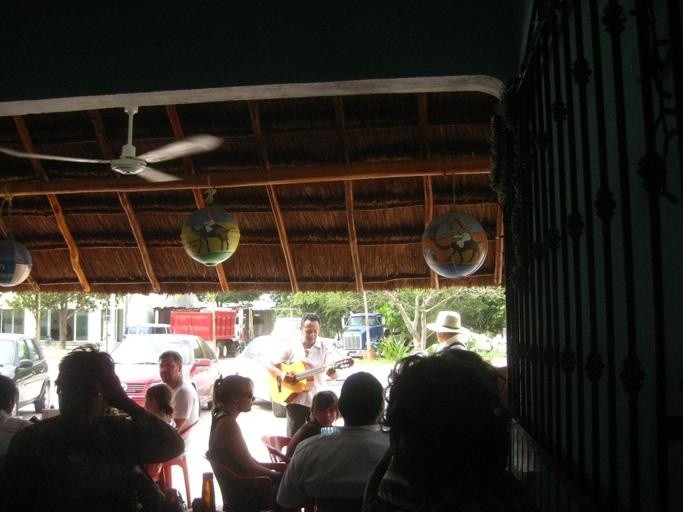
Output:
<box><xmin>146</xmin><ymin>418</ymin><xmax>293</xmax><ymax>509</ymax></box>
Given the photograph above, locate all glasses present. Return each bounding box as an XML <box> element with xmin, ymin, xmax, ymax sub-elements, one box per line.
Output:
<box><xmin>57</xmin><ymin>387</ymin><xmax>102</xmax><ymax>399</ymax></box>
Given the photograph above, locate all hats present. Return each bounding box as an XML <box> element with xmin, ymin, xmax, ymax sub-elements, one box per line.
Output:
<box><xmin>426</xmin><ymin>311</ymin><xmax>467</xmax><ymax>332</ymax></box>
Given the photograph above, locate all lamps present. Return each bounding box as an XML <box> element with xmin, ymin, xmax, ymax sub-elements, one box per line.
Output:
<box><xmin>180</xmin><ymin>188</ymin><xmax>241</xmax><ymax>267</ymax></box>
<box><xmin>0</xmin><ymin>192</ymin><xmax>33</xmax><ymax>287</ymax></box>
<box><xmin>420</xmin><ymin>169</ymin><xmax>489</xmax><ymax>278</ymax></box>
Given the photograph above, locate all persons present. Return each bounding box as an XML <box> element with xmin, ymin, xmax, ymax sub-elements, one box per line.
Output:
<box><xmin>422</xmin><ymin>310</ymin><xmax>471</xmax><ymax>352</ymax></box>
<box><xmin>275</xmin><ymin>370</ymin><xmax>395</xmax><ymax>512</ymax></box>
<box><xmin>207</xmin><ymin>371</ymin><xmax>285</xmax><ymax>512</ymax></box>
<box><xmin>1</xmin><ymin>341</ymin><xmax>188</xmax><ymax>511</ymax></box>
<box><xmin>375</xmin><ymin>345</ymin><xmax>540</xmax><ymax>511</ymax></box>
<box><xmin>0</xmin><ymin>375</ymin><xmax>38</xmax><ymax>465</ymax></box>
<box><xmin>284</xmin><ymin>390</ymin><xmax>343</xmax><ymax>459</ymax></box>
<box><xmin>266</xmin><ymin>312</ymin><xmax>342</xmax><ymax>438</ymax></box>
<box><xmin>134</xmin><ymin>383</ymin><xmax>177</xmax><ymax>491</ymax></box>
<box><xmin>157</xmin><ymin>349</ymin><xmax>201</xmax><ymax>446</ymax></box>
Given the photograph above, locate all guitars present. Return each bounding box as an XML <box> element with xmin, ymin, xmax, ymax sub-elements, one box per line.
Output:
<box><xmin>269</xmin><ymin>357</ymin><xmax>353</xmax><ymax>405</ymax></box>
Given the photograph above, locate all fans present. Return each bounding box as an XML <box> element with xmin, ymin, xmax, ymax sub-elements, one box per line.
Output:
<box><xmin>0</xmin><ymin>104</ymin><xmax>224</xmax><ymax>182</ymax></box>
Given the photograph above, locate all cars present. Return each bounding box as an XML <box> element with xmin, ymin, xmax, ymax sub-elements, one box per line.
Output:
<box><xmin>99</xmin><ymin>333</ymin><xmax>221</xmax><ymax>417</ymax></box>
<box><xmin>1</xmin><ymin>333</ymin><xmax>52</xmax><ymax>420</ymax></box>
<box><xmin>242</xmin><ymin>336</ymin><xmax>365</xmax><ymax>418</ymax></box>
<box><xmin>121</xmin><ymin>324</ymin><xmax>171</xmax><ymax>341</ymax></box>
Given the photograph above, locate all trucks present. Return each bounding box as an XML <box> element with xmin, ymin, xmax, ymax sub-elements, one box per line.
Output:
<box><xmin>170</xmin><ymin>307</ymin><xmax>247</xmax><ymax>358</ymax></box>
<box><xmin>334</xmin><ymin>309</ymin><xmax>408</xmax><ymax>354</ymax></box>
